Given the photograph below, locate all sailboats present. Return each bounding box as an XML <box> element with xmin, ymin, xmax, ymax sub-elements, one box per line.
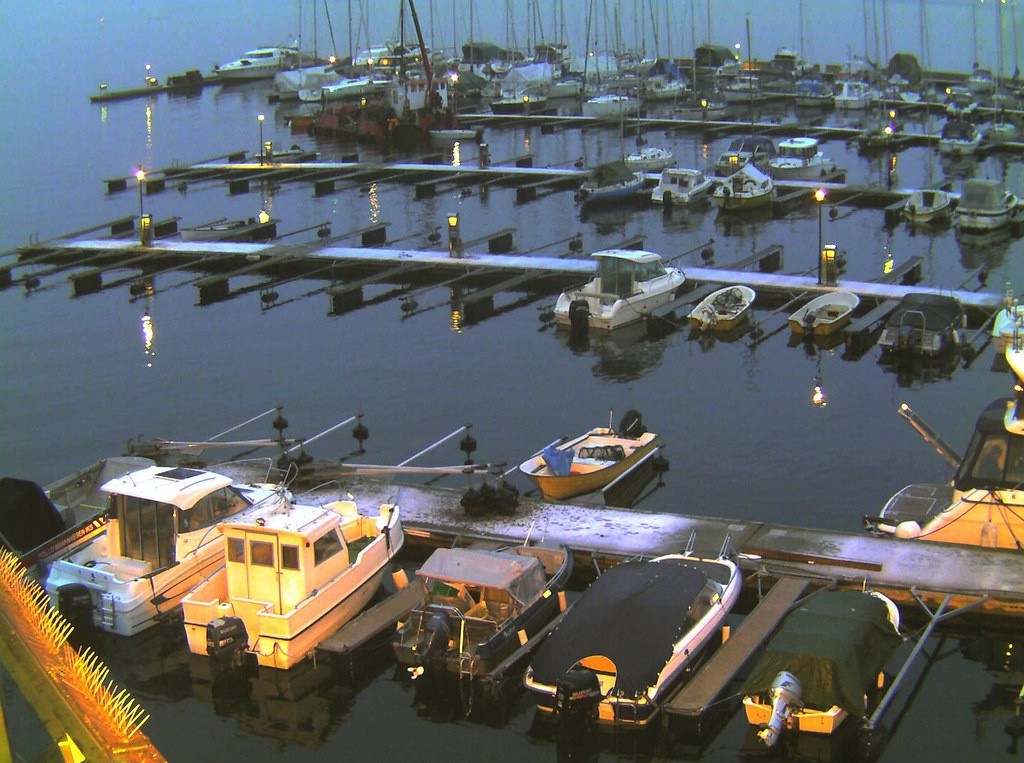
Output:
<box><xmin>903</xmin><ymin>0</ymin><xmax>950</xmax><ymax>222</ymax></box>
<box><xmin>267</xmin><ymin>0</ymin><xmax>1024</xmax><ymax>206</ymax></box>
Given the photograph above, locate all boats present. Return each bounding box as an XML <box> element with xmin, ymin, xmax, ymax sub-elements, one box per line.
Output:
<box><xmin>788</xmin><ymin>290</ymin><xmax>862</xmax><ymax>335</ymax></box>
<box><xmin>685</xmin><ymin>285</ymin><xmax>756</xmax><ymax>331</ymax></box>
<box><xmin>955</xmin><ymin>226</ymin><xmax>1024</xmax><ymax>271</ymax></box>
<box><xmin>520</xmin><ymin>405</ymin><xmax>660</xmax><ymax>502</ymax></box>
<box><xmin>742</xmin><ymin>581</ymin><xmax>901</xmax><ymax>755</ymax></box>
<box><xmin>522</xmin><ymin>527</ymin><xmax>744</xmax><ymax>734</ymax></box>
<box><xmin>862</xmin><ymin>278</ymin><xmax>1023</xmax><ymax>616</ymax></box>
<box><xmin>875</xmin><ymin>294</ymin><xmax>964</xmax><ymax>359</ymax></box>
<box><xmin>553</xmin><ymin>247</ymin><xmax>685</xmax><ymax>329</ymax></box>
<box><xmin>389</xmin><ymin>535</ymin><xmax>573</xmax><ymax>680</ymax></box>
<box><xmin>45</xmin><ymin>457</ymin><xmax>300</xmax><ymax>638</ymax></box>
<box><xmin>956</xmin><ymin>179</ymin><xmax>1019</xmax><ymax>230</ymax></box>
<box><xmin>214</xmin><ymin>32</ymin><xmax>317</xmax><ymax>81</ymax></box>
<box><xmin>179</xmin><ymin>480</ymin><xmax>404</xmax><ymax>670</ymax></box>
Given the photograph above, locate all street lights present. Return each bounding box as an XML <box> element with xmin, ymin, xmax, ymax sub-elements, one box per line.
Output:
<box><xmin>885</xmin><ymin>127</ymin><xmax>893</xmax><ymax>190</ymax></box>
<box><xmin>258</xmin><ymin>114</ymin><xmax>264</xmax><ymax>167</ymax></box>
<box><xmin>146</xmin><ymin>64</ymin><xmax>150</xmax><ymax>87</ymax></box>
<box><xmin>816</xmin><ymin>189</ymin><xmax>825</xmax><ymax>284</ymax></box>
